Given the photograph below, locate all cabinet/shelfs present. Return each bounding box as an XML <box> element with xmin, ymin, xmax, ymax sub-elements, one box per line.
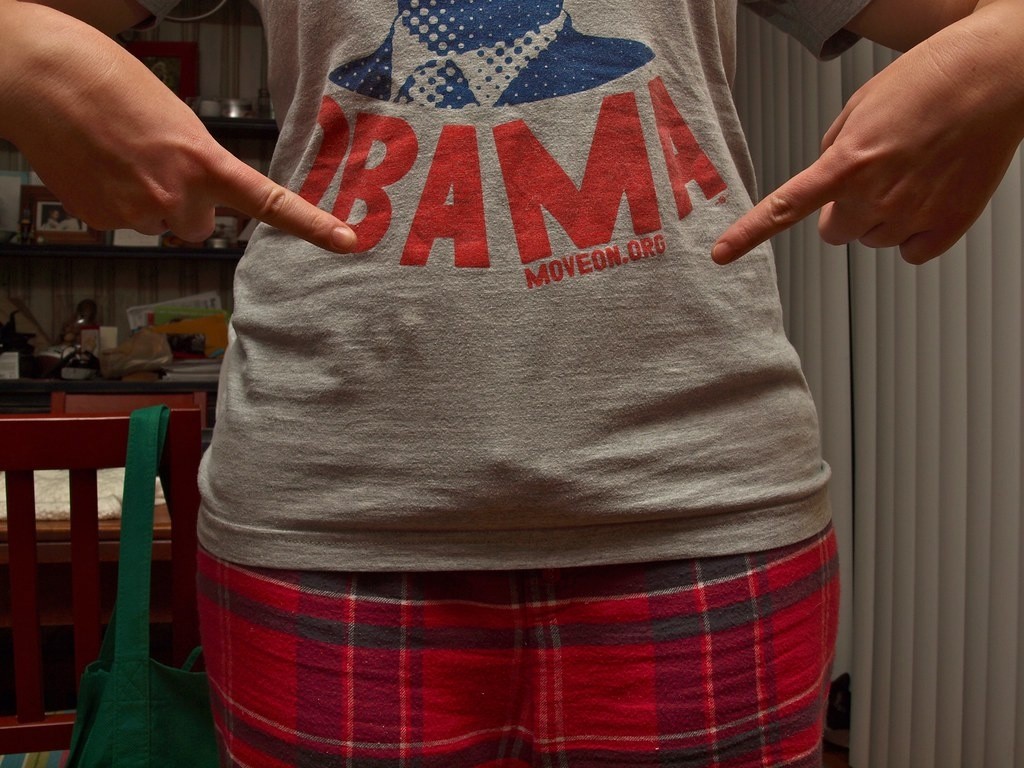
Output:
<box><xmin>0</xmin><ymin>119</ymin><xmax>278</xmax><ymax>259</ymax></box>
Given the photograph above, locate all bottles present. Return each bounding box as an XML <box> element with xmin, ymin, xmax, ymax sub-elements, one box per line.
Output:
<box><xmin>20</xmin><ymin>203</ymin><xmax>32</xmax><ymax>244</ymax></box>
<box><xmin>258</xmin><ymin>89</ymin><xmax>270</xmax><ymax>119</ymax></box>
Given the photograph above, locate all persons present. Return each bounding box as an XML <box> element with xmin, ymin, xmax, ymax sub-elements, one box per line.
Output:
<box><xmin>2</xmin><ymin>0</ymin><xmax>1022</xmax><ymax>768</ymax></box>
<box><xmin>42</xmin><ymin>210</ymin><xmax>86</xmax><ymax>232</ymax></box>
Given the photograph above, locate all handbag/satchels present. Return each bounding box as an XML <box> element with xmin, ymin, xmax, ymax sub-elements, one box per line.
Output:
<box><xmin>64</xmin><ymin>403</ymin><xmax>221</xmax><ymax>768</ymax></box>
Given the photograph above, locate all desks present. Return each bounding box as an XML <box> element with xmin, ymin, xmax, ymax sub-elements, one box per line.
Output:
<box><xmin>0</xmin><ymin>500</ymin><xmax>172</xmax><ymax>565</ymax></box>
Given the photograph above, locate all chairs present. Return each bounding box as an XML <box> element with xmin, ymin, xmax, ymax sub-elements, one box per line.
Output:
<box><xmin>0</xmin><ymin>389</ymin><xmax>206</xmax><ymax>768</ymax></box>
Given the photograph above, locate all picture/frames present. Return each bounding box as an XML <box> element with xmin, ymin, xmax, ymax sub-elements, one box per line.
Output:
<box><xmin>182</xmin><ymin>208</ymin><xmax>243</xmax><ymax>247</ymax></box>
<box><xmin>125</xmin><ymin>39</ymin><xmax>198</xmax><ymax>107</ymax></box>
<box><xmin>18</xmin><ymin>185</ymin><xmax>106</xmax><ymax>245</ymax></box>
